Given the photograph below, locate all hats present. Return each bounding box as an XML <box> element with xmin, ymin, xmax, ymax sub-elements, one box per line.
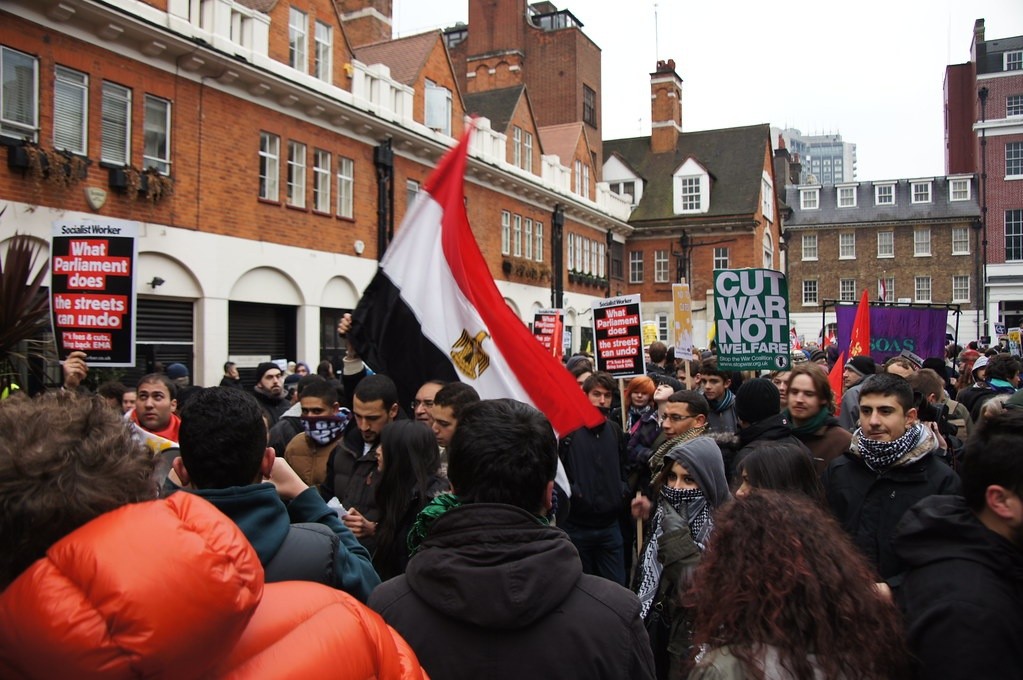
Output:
<box><xmin>168</xmin><ymin>363</ymin><xmax>189</xmax><ymax>378</ymax></box>
<box><xmin>735</xmin><ymin>379</ymin><xmax>781</xmax><ymax>422</ymax></box>
<box><xmin>809</xmin><ymin>347</ymin><xmax>826</xmax><ymax>362</ymax></box>
<box><xmin>844</xmin><ymin>356</ymin><xmax>876</xmax><ymax>377</ymax></box>
<box><xmin>257</xmin><ymin>362</ymin><xmax>283</xmax><ymax>383</ymax></box>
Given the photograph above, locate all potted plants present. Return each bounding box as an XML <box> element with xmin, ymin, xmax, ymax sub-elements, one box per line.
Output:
<box><xmin>567</xmin><ymin>268</ymin><xmax>609</xmax><ymax>291</ymax></box>
<box><xmin>139</xmin><ymin>171</ymin><xmax>162</xmax><ymax>216</ymax></box>
<box><xmin>108</xmin><ymin>163</ymin><xmax>144</xmax><ymax>221</ymax></box>
<box><xmin>55</xmin><ymin>147</ymin><xmax>83</xmax><ymax>199</ymax></box>
<box><xmin>37</xmin><ymin>146</ymin><xmax>69</xmax><ymax>217</ymax></box>
<box><xmin>501</xmin><ymin>257</ymin><xmax>553</xmax><ymax>282</ymax></box>
<box><xmin>154</xmin><ymin>172</ymin><xmax>179</xmax><ymax>210</ymax></box>
<box><xmin>7</xmin><ymin>141</ymin><xmax>45</xmax><ymax>217</ymax></box>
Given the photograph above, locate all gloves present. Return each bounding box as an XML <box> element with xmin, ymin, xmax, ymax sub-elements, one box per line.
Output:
<box><xmin>660</xmin><ymin>500</ymin><xmax>689</xmax><ymax>534</ymax></box>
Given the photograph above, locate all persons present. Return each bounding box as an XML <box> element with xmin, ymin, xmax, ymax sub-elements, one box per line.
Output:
<box><xmin>0</xmin><ymin>312</ymin><xmax>1023</xmax><ymax>680</ymax></box>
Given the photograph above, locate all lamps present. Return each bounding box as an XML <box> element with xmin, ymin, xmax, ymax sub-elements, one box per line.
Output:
<box><xmin>147</xmin><ymin>273</ymin><xmax>166</xmax><ymax>289</ymax></box>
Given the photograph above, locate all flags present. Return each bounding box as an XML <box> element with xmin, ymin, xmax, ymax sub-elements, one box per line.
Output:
<box><xmin>341</xmin><ymin>129</ymin><xmax>603</xmax><ymax>500</ymax></box>
<box><xmin>827</xmin><ymin>354</ymin><xmax>843</xmax><ymax>417</ymax></box>
<box><xmin>848</xmin><ymin>290</ymin><xmax>871</xmax><ymax>360</ymax></box>
<box><xmin>879</xmin><ymin>277</ymin><xmax>887</xmax><ymax>305</ymax></box>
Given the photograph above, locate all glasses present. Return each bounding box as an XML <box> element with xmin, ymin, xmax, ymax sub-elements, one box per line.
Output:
<box><xmin>661</xmin><ymin>414</ymin><xmax>693</xmax><ymax>422</ymax></box>
<box><xmin>410</xmin><ymin>400</ymin><xmax>434</xmax><ymax>409</ymax></box>
